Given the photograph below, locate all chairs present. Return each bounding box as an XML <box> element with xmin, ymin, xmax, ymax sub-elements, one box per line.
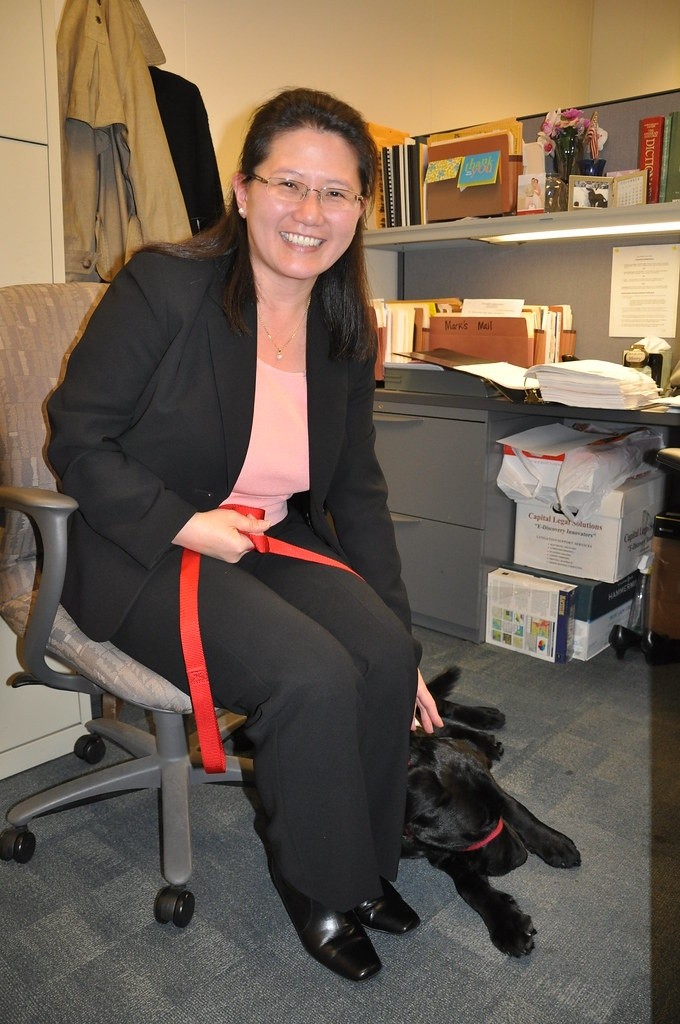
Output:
<box><xmin>0</xmin><ymin>281</ymin><xmax>259</xmax><ymax>934</ymax></box>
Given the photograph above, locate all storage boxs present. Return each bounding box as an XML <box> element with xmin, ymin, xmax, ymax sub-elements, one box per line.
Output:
<box><xmin>512</xmin><ymin>471</ymin><xmax>668</xmax><ymax>584</ymax></box>
<box><xmin>485</xmin><ymin>568</ymin><xmax>578</xmax><ymax>665</ymax></box>
<box><xmin>501</xmin><ymin>426</ymin><xmax>663</xmax><ymax>492</ymax></box>
<box><xmin>507</xmin><ymin>565</ymin><xmax>643</xmax><ymax>662</ymax></box>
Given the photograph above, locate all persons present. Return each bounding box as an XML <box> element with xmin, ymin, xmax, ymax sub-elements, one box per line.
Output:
<box><xmin>525</xmin><ymin>177</ymin><xmax>541</xmax><ymax>210</ymax></box>
<box><xmin>45</xmin><ymin>86</ymin><xmax>443</xmax><ymax>982</ymax></box>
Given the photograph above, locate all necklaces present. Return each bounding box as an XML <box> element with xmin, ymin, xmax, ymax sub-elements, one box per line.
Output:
<box><xmin>255</xmin><ymin>297</ymin><xmax>311</xmax><ymax>360</ymax></box>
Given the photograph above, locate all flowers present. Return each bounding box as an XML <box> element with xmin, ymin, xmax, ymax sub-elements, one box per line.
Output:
<box><xmin>535</xmin><ymin>107</ymin><xmax>609</xmax><ymax>179</ymax></box>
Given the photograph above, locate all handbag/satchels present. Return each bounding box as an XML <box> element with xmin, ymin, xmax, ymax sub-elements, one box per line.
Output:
<box><xmin>497</xmin><ymin>428</ymin><xmax>664</xmax><ymax>524</ymax></box>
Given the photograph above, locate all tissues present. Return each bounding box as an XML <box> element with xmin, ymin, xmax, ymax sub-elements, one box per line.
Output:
<box><xmin>623</xmin><ymin>335</ymin><xmax>673</xmax><ymax>396</ymax></box>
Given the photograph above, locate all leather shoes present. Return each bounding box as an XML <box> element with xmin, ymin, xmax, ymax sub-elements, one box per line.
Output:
<box><xmin>271</xmin><ymin>850</ymin><xmax>384</xmax><ymax>983</ymax></box>
<box><xmin>342</xmin><ymin>873</ymin><xmax>423</xmax><ymax>937</ymax></box>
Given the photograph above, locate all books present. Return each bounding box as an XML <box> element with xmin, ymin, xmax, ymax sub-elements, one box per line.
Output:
<box><xmin>361</xmin><ymin>122</ymin><xmax>523</xmax><ymax>230</ymax></box>
<box><xmin>638</xmin><ymin>111</ymin><xmax>680</xmax><ymax>203</ymax></box>
<box><xmin>368</xmin><ymin>296</ymin><xmax>572</xmax><ymax>382</ymax></box>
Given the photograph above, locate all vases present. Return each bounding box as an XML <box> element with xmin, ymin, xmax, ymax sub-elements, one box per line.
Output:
<box><xmin>552</xmin><ymin>129</ymin><xmax>583</xmax><ymax>189</ymax></box>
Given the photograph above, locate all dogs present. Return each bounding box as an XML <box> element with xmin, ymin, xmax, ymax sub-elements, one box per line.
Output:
<box><xmin>387</xmin><ymin>664</ymin><xmax>583</xmax><ymax>958</ymax></box>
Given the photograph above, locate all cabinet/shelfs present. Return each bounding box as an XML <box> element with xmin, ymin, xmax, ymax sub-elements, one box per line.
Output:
<box><xmin>370</xmin><ymin>387</ymin><xmax>680</xmax><ymax>645</ymax></box>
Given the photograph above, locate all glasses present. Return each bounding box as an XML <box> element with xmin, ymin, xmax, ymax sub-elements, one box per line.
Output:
<box><xmin>241</xmin><ymin>170</ymin><xmax>364</xmax><ymax>212</ymax></box>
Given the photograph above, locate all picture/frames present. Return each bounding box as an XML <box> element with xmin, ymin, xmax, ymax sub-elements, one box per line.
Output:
<box><xmin>568</xmin><ymin>175</ymin><xmax>613</xmax><ymax>211</ymax></box>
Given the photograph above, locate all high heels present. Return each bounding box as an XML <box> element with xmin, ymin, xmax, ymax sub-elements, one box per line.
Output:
<box><xmin>640</xmin><ymin>628</ymin><xmax>680</xmax><ymax>664</ymax></box>
<box><xmin>608</xmin><ymin>625</ymin><xmax>642</xmax><ymax>660</ymax></box>
